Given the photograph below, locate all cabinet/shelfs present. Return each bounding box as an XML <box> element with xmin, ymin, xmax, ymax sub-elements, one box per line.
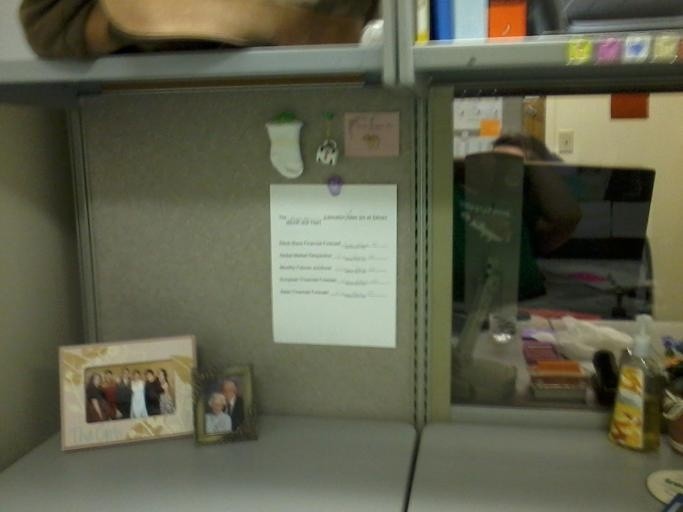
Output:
<box><xmin>2</xmin><ymin>6</ymin><xmax>683</xmax><ymax>508</ymax></box>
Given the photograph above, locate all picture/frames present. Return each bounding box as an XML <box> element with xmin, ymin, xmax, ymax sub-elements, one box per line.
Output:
<box><xmin>57</xmin><ymin>334</ymin><xmax>198</xmax><ymax>452</ymax></box>
<box><xmin>191</xmin><ymin>364</ymin><xmax>259</xmax><ymax>444</ymax></box>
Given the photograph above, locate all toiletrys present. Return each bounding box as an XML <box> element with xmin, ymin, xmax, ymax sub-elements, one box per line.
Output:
<box><xmin>609</xmin><ymin>313</ymin><xmax>664</xmax><ymax>453</ymax></box>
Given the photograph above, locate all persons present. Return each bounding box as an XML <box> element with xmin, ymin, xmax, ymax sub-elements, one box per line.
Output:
<box><xmin>217</xmin><ymin>381</ymin><xmax>245</xmax><ymax>430</ymax></box>
<box><xmin>491</xmin><ymin>129</ymin><xmax>567</xmax><ymax>164</ymax></box>
<box><xmin>86</xmin><ymin>366</ymin><xmax>174</xmax><ymax>423</ymax></box>
<box><xmin>205</xmin><ymin>392</ymin><xmax>232</xmax><ymax>435</ymax></box>
